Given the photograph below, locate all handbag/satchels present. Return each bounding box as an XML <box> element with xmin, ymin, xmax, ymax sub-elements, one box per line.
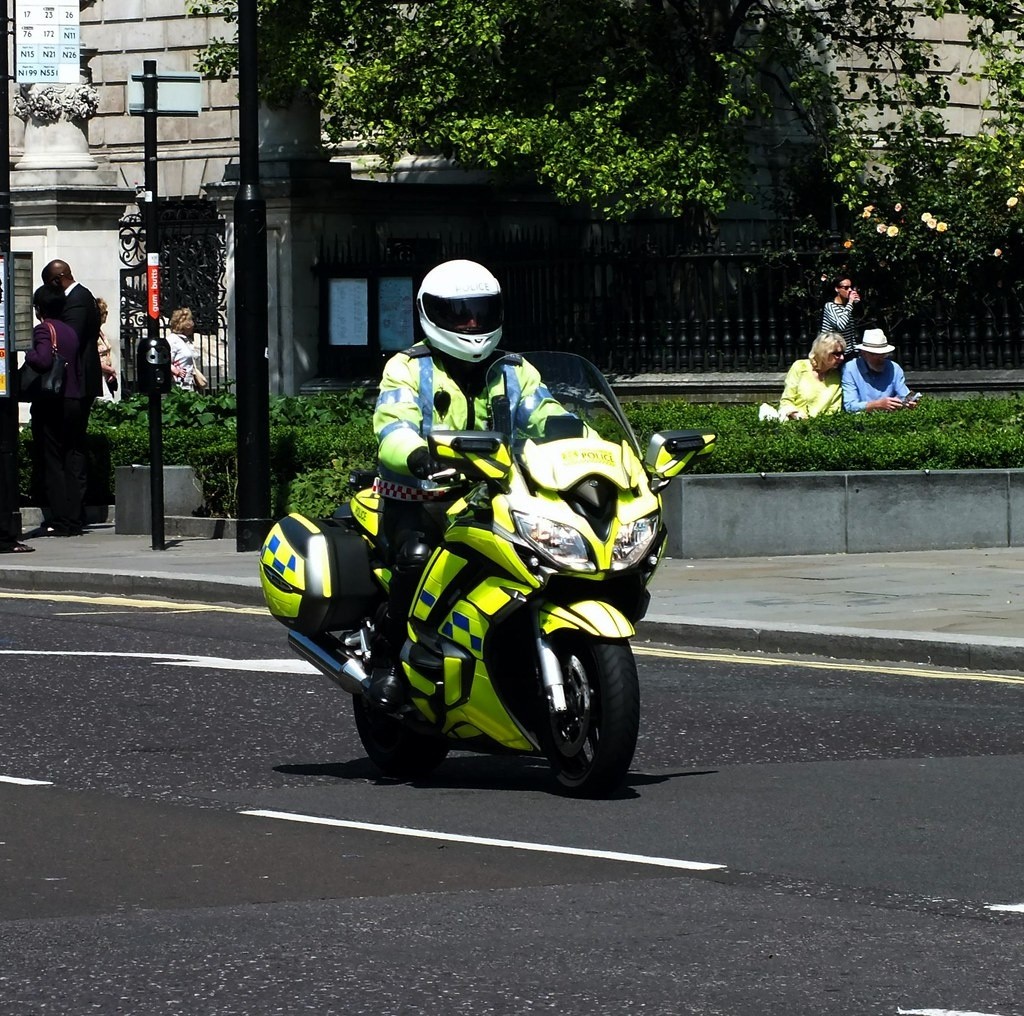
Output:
<box><xmin>15</xmin><ymin>322</ymin><xmax>69</xmax><ymax>403</ymax></box>
<box><xmin>194</xmin><ymin>368</ymin><xmax>208</xmax><ymax>388</ymax></box>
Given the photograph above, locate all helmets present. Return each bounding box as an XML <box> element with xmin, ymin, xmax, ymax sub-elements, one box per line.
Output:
<box><xmin>415</xmin><ymin>259</ymin><xmax>504</xmax><ymax>362</ymax></box>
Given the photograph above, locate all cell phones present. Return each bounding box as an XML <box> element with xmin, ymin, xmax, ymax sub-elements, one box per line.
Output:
<box><xmin>912</xmin><ymin>392</ymin><xmax>922</xmax><ymax>402</ymax></box>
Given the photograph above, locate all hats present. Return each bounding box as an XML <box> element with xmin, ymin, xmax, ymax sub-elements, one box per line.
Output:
<box><xmin>854</xmin><ymin>328</ymin><xmax>895</xmax><ymax>353</ymax></box>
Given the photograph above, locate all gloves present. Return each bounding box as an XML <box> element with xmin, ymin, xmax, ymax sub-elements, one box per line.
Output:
<box><xmin>407</xmin><ymin>447</ymin><xmax>450</xmax><ymax>484</ymax></box>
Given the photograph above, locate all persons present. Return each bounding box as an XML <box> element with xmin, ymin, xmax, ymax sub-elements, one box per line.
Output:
<box><xmin>842</xmin><ymin>328</ymin><xmax>921</xmax><ymax>411</ymax></box>
<box><xmin>366</xmin><ymin>260</ymin><xmax>601</xmax><ymax>711</ymax></box>
<box><xmin>165</xmin><ymin>307</ymin><xmax>205</xmax><ymax>394</ymax></box>
<box><xmin>758</xmin><ymin>333</ymin><xmax>846</xmax><ymax>422</ymax></box>
<box><xmin>0</xmin><ymin>261</ymin><xmax>117</xmax><ymax>553</ymax></box>
<box><xmin>821</xmin><ymin>275</ymin><xmax>862</xmax><ymax>365</ymax></box>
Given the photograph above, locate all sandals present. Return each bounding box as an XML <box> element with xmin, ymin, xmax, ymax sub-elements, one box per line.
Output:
<box><xmin>0</xmin><ymin>540</ymin><xmax>34</xmax><ymax>552</ymax></box>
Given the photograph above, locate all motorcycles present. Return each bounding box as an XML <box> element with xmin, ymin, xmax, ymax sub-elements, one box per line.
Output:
<box><xmin>261</xmin><ymin>350</ymin><xmax>718</xmax><ymax>796</ymax></box>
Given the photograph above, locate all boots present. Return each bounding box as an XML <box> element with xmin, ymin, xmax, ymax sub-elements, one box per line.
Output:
<box><xmin>368</xmin><ymin>612</ymin><xmax>407</xmax><ymax>711</ymax></box>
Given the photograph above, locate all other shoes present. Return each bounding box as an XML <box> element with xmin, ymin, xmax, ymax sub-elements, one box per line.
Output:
<box><xmin>37</xmin><ymin>523</ymin><xmax>81</xmax><ymax>537</ymax></box>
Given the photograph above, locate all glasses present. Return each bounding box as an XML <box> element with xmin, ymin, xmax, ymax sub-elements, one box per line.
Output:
<box><xmin>830</xmin><ymin>351</ymin><xmax>845</xmax><ymax>356</ymax></box>
<box><xmin>838</xmin><ymin>285</ymin><xmax>852</xmax><ymax>290</ymax></box>
